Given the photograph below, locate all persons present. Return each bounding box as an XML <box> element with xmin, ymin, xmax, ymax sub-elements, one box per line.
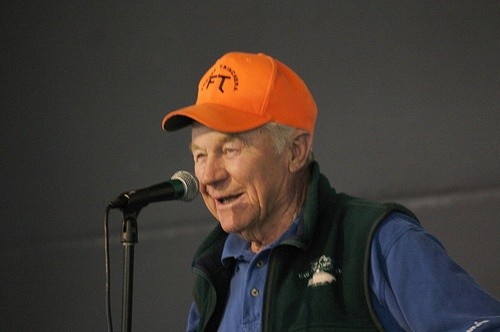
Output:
<box><xmin>162</xmin><ymin>50</ymin><xmax>500</xmax><ymax>332</ymax></box>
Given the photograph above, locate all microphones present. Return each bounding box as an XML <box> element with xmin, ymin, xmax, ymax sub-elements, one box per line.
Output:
<box><xmin>108</xmin><ymin>169</ymin><xmax>200</xmax><ymax>209</ymax></box>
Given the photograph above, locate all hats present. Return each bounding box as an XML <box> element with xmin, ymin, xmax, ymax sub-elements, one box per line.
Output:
<box><xmin>162</xmin><ymin>52</ymin><xmax>317</xmax><ymax>138</ymax></box>
